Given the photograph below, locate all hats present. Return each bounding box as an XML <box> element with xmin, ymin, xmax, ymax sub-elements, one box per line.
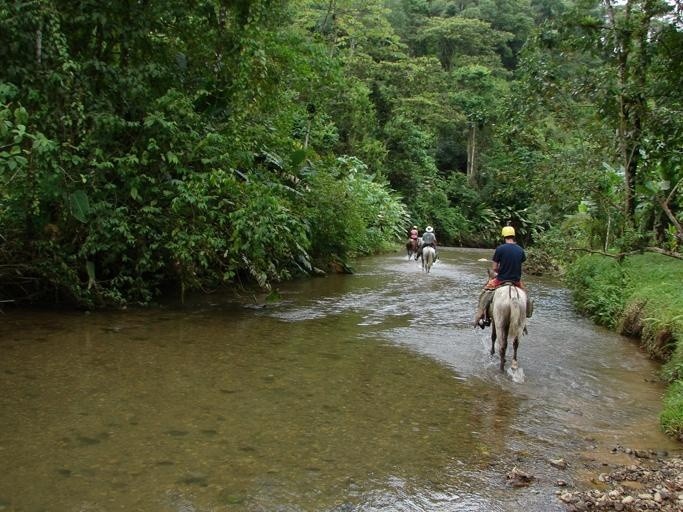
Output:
<box><xmin>425</xmin><ymin>226</ymin><xmax>433</xmax><ymax>232</ymax></box>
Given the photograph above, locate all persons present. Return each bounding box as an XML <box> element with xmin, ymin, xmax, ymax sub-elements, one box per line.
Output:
<box><xmin>477</xmin><ymin>226</ymin><xmax>526</xmax><ymax>326</ymax></box>
<box><xmin>415</xmin><ymin>226</ymin><xmax>437</xmax><ymax>260</ymax></box>
<box><xmin>411</xmin><ymin>226</ymin><xmax>418</xmax><ymax>241</ymax></box>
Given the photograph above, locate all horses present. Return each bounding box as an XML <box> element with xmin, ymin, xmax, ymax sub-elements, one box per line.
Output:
<box><xmin>416</xmin><ymin>239</ymin><xmax>436</xmax><ymax>273</ymax></box>
<box><xmin>487</xmin><ymin>265</ymin><xmax>527</xmax><ymax>374</ymax></box>
<box><xmin>406</xmin><ymin>238</ymin><xmax>418</xmax><ymax>260</ymax></box>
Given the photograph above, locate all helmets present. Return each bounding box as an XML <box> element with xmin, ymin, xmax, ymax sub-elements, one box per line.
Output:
<box><xmin>502</xmin><ymin>226</ymin><xmax>516</xmax><ymax>238</ymax></box>
